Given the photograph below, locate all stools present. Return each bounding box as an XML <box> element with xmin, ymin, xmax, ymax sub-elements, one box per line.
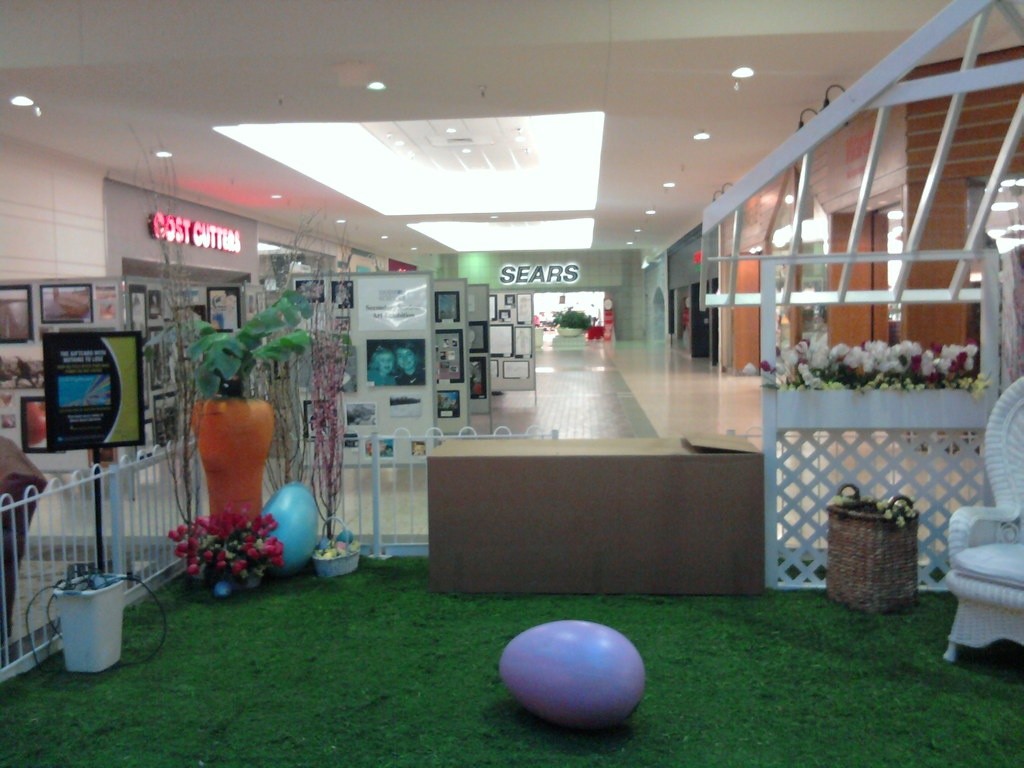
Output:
<box><xmin>588</xmin><ymin>327</ymin><xmax>604</xmax><ymax>340</ymax></box>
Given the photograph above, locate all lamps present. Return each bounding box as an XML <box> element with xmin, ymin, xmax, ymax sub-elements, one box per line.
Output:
<box><xmin>820</xmin><ymin>84</ymin><xmax>846</xmax><ymax>112</ymax></box>
<box><xmin>990</xmin><ymin>187</ymin><xmax>1019</xmax><ymax>211</ymax></box>
<box><xmin>794</xmin><ymin>108</ymin><xmax>818</xmax><ymax>133</ymax></box>
<box><xmin>985</xmin><ymin>209</ymin><xmax>1010</xmax><ymax>238</ymax></box>
<box><xmin>640</xmin><ymin>256</ymin><xmax>662</xmax><ymax>270</ymax></box>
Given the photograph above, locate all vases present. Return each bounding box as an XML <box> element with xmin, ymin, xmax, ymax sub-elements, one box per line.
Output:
<box><xmin>216</xmin><ymin>568</ymin><xmax>264</xmax><ymax>590</ymax></box>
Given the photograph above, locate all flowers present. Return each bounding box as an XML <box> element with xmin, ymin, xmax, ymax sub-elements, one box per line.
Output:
<box><xmin>744</xmin><ymin>336</ymin><xmax>993</xmax><ymax>402</ymax></box>
<box><xmin>168</xmin><ymin>501</ymin><xmax>285</xmax><ymax>580</ymax></box>
<box><xmin>834</xmin><ymin>495</ymin><xmax>916</xmax><ymax>529</ymax></box>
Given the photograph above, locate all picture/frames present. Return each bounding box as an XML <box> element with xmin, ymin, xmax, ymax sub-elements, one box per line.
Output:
<box><xmin>490</xmin><ymin>323</ymin><xmax>514</xmax><ymax>358</ymax></box>
<box><xmin>147</xmin><ymin>290</ymin><xmax>161</xmax><ymax>315</ymax></box>
<box><xmin>345</xmin><ymin>402</ymin><xmax>378</xmax><ymax>428</ymax></box>
<box><xmin>128</xmin><ymin>283</ymin><xmax>148</xmax><ymax>344</ymax></box>
<box><xmin>40</xmin><ymin>284</ymin><xmax>93</xmax><ymax>324</ymax></box>
<box><xmin>303</xmin><ymin>399</ymin><xmax>337</xmax><ymax>439</ymax></box>
<box><xmin>274</xmin><ymin>360</ymin><xmax>281</xmax><ymax>380</ymax></box>
<box><xmin>499</xmin><ymin>310</ymin><xmax>511</xmax><ymax>319</ymax></box>
<box><xmin>469</xmin><ymin>356</ymin><xmax>487</xmax><ymax>400</ymax></box>
<box><xmin>490</xmin><ymin>360</ymin><xmax>499</xmax><ymax>377</ymax></box>
<box><xmin>514</xmin><ymin>325</ymin><xmax>533</xmax><ymax>359</ymax></box>
<box><xmin>334</xmin><ymin>316</ymin><xmax>351</xmax><ymax>333</ymax></box>
<box><xmin>489</xmin><ymin>294</ymin><xmax>498</xmax><ymax>321</ymax></box>
<box><xmin>20</xmin><ymin>396</ymin><xmax>54</xmax><ymax>454</ymax></box>
<box><xmin>153</xmin><ymin>391</ymin><xmax>179</xmax><ymax>447</ymax></box>
<box><xmin>207</xmin><ymin>287</ymin><xmax>241</xmax><ymax>333</ymax></box>
<box><xmin>331</xmin><ymin>280</ymin><xmax>354</xmax><ymax>309</ymax></box>
<box><xmin>437</xmin><ymin>390</ymin><xmax>461</xmax><ymax>418</ymax></box>
<box><xmin>147</xmin><ymin>326</ymin><xmax>164</xmax><ymax>390</ymax></box>
<box><xmin>296</xmin><ymin>280</ymin><xmax>325</xmax><ymax>303</ymax></box>
<box><xmin>469</xmin><ymin>321</ymin><xmax>488</xmax><ymax>353</ymax></box>
<box><xmin>434</xmin><ymin>329</ymin><xmax>464</xmax><ymax>384</ymax></box>
<box><xmin>503</xmin><ymin>361</ymin><xmax>530</xmax><ymax>379</ymax></box>
<box><xmin>0</xmin><ymin>284</ymin><xmax>35</xmax><ymax>345</ymax></box>
<box><xmin>434</xmin><ymin>291</ymin><xmax>460</xmax><ymax>322</ymax></box>
<box><xmin>504</xmin><ymin>295</ymin><xmax>516</xmax><ymax>305</ymax></box>
<box><xmin>363</xmin><ymin>435</ymin><xmax>396</xmax><ymax>461</ymax></box>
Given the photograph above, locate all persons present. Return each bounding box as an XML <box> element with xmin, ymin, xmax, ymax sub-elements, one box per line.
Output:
<box><xmin>393</xmin><ymin>343</ymin><xmax>426</xmax><ymax>386</ymax></box>
<box><xmin>10</xmin><ymin>356</ymin><xmax>37</xmax><ymax>388</ymax></box>
<box><xmin>367</xmin><ymin>346</ymin><xmax>398</xmax><ymax>386</ymax></box>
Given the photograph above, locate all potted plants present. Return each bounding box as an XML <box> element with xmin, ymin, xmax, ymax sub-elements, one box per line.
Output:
<box><xmin>552</xmin><ymin>307</ymin><xmax>592</xmax><ymax>338</ymax></box>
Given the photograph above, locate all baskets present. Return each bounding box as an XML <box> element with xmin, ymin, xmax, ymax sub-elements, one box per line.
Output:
<box><xmin>825</xmin><ymin>483</ymin><xmax>919</xmax><ymax>613</ymax></box>
<box><xmin>313</xmin><ymin>516</ymin><xmax>361</xmax><ymax>578</ymax></box>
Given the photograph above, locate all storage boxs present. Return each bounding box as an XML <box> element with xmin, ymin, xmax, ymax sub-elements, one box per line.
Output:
<box><xmin>427</xmin><ymin>438</ymin><xmax>765</xmax><ymax>599</ymax></box>
<box><xmin>52</xmin><ymin>572</ymin><xmax>130</xmax><ymax>674</ymax></box>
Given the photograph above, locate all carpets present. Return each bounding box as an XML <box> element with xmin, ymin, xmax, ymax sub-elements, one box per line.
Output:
<box><xmin>0</xmin><ymin>554</ymin><xmax>1024</xmax><ymax>768</ymax></box>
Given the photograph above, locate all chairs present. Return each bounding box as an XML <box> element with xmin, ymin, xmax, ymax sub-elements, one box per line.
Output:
<box><xmin>941</xmin><ymin>375</ymin><xmax>1024</xmax><ymax>666</ymax></box>
